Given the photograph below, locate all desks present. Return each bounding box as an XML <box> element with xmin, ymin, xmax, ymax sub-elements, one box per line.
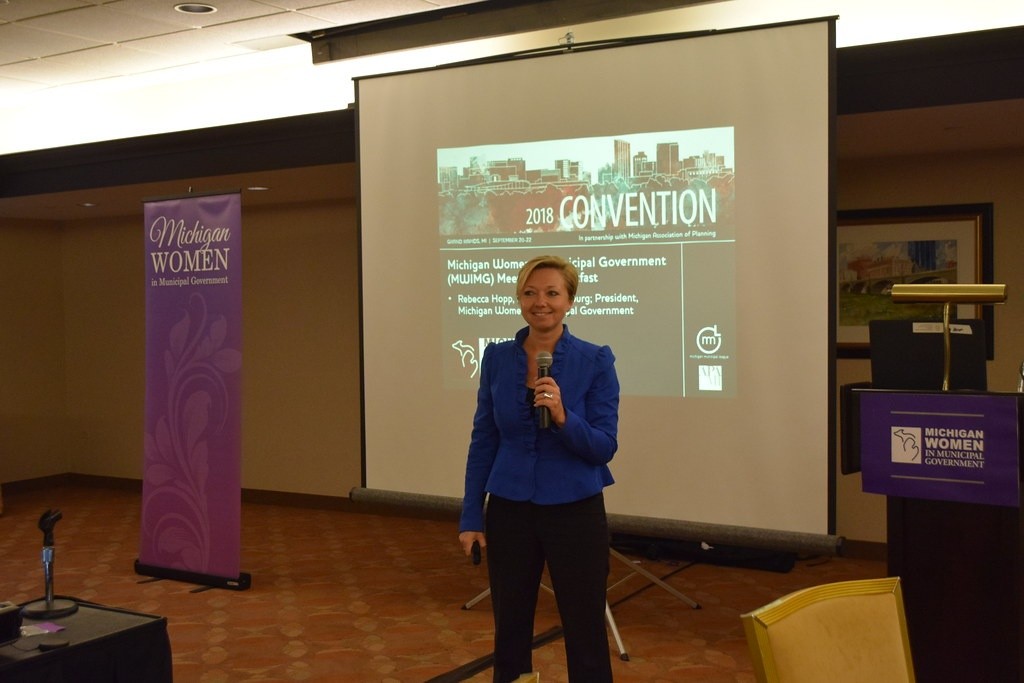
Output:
<box><xmin>0</xmin><ymin>594</ymin><xmax>176</xmax><ymax>683</ymax></box>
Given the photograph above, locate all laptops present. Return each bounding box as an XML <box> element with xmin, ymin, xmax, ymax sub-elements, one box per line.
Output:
<box><xmin>870</xmin><ymin>318</ymin><xmax>987</xmax><ymax>391</ymax></box>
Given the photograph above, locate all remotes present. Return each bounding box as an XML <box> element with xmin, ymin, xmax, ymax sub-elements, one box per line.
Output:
<box><xmin>473</xmin><ymin>541</ymin><xmax>481</xmax><ymax>565</ymax></box>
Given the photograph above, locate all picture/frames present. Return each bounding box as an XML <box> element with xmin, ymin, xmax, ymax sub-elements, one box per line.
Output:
<box><xmin>837</xmin><ymin>201</ymin><xmax>994</xmax><ymax>360</ymax></box>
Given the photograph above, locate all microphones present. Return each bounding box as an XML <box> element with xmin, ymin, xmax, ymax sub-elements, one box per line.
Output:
<box><xmin>536</xmin><ymin>352</ymin><xmax>553</xmax><ymax>427</ymax></box>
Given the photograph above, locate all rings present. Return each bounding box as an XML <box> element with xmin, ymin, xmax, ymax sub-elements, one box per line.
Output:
<box><xmin>544</xmin><ymin>391</ymin><xmax>553</xmax><ymax>398</ymax></box>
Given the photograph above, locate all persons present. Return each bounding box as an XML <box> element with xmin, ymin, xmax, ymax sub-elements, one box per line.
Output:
<box><xmin>457</xmin><ymin>256</ymin><xmax>620</xmax><ymax>683</ymax></box>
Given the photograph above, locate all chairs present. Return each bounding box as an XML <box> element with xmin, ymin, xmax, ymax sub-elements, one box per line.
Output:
<box><xmin>739</xmin><ymin>575</ymin><xmax>917</xmax><ymax>683</ymax></box>
